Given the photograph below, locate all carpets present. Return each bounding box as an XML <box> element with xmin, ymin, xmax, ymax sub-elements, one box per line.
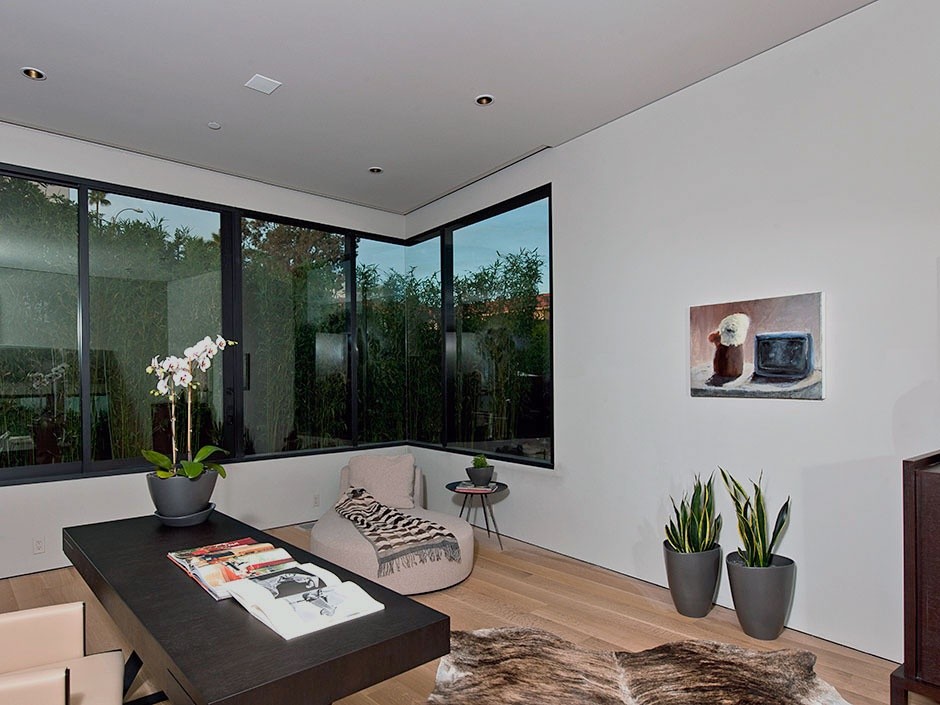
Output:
<box><xmin>425</xmin><ymin>624</ymin><xmax>850</xmax><ymax>705</ymax></box>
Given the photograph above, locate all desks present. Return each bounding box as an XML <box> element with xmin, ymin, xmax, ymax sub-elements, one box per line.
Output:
<box><xmin>443</xmin><ymin>480</ymin><xmax>509</xmax><ymax>553</ymax></box>
<box><xmin>61</xmin><ymin>508</ymin><xmax>454</xmax><ymax>705</ymax></box>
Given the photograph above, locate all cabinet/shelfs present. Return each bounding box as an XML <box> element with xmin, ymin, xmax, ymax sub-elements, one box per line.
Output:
<box><xmin>889</xmin><ymin>448</ymin><xmax>940</xmax><ymax>705</ymax></box>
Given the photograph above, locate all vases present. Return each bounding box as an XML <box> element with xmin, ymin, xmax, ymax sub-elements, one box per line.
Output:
<box><xmin>146</xmin><ymin>469</ymin><xmax>220</xmax><ymax>527</ymax></box>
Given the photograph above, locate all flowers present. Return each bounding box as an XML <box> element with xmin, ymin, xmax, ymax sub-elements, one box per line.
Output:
<box><xmin>142</xmin><ymin>334</ymin><xmax>240</xmax><ymax>480</ymax></box>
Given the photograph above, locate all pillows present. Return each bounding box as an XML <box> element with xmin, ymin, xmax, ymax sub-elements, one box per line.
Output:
<box><xmin>347</xmin><ymin>453</ymin><xmax>416</xmax><ymax>509</ymax></box>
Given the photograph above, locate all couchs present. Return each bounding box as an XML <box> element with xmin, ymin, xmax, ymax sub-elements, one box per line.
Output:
<box><xmin>308</xmin><ymin>452</ymin><xmax>475</xmax><ymax>597</ymax></box>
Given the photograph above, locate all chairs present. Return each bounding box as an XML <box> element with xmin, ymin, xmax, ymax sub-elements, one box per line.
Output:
<box><xmin>0</xmin><ymin>600</ymin><xmax>157</xmax><ymax>705</ymax></box>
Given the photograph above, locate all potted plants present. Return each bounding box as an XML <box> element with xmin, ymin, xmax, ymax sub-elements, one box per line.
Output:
<box><xmin>662</xmin><ymin>464</ymin><xmax>798</xmax><ymax>641</ymax></box>
<box><xmin>463</xmin><ymin>456</ymin><xmax>497</xmax><ymax>486</ymax></box>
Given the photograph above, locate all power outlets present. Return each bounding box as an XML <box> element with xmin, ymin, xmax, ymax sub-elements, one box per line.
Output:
<box><xmin>32</xmin><ymin>536</ymin><xmax>45</xmax><ymax>554</ymax></box>
